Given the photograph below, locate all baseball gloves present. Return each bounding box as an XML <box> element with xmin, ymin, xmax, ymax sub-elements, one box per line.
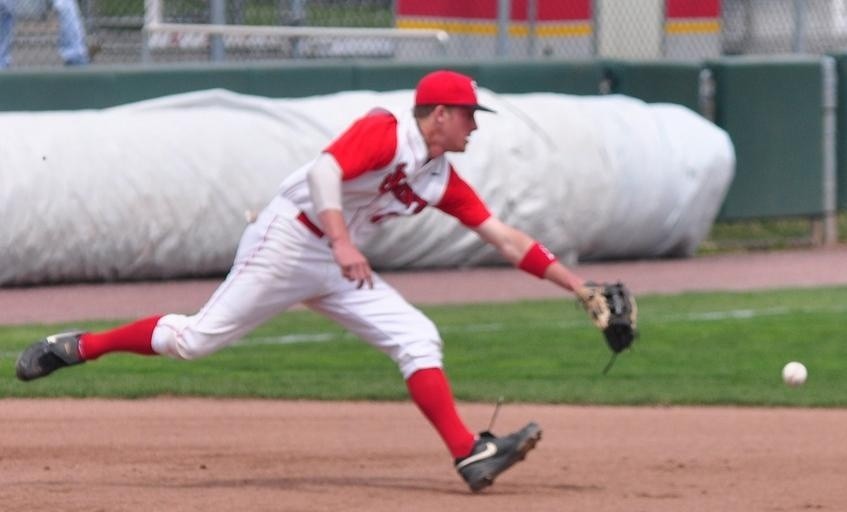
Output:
<box><xmin>575</xmin><ymin>280</ymin><xmax>637</xmax><ymax>375</ymax></box>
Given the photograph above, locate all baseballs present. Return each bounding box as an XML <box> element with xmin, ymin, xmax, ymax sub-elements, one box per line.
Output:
<box><xmin>783</xmin><ymin>362</ymin><xmax>807</xmax><ymax>386</ymax></box>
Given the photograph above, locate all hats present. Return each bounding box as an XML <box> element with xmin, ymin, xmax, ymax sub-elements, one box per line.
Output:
<box><xmin>416</xmin><ymin>71</ymin><xmax>497</xmax><ymax>113</ymax></box>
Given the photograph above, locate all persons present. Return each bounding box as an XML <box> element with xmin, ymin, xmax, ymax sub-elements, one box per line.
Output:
<box><xmin>16</xmin><ymin>70</ymin><xmax>611</xmax><ymax>493</ymax></box>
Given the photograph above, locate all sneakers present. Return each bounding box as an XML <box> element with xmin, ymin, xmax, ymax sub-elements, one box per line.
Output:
<box><xmin>15</xmin><ymin>331</ymin><xmax>85</xmax><ymax>381</ymax></box>
<box><xmin>454</xmin><ymin>422</ymin><xmax>544</xmax><ymax>492</ymax></box>
